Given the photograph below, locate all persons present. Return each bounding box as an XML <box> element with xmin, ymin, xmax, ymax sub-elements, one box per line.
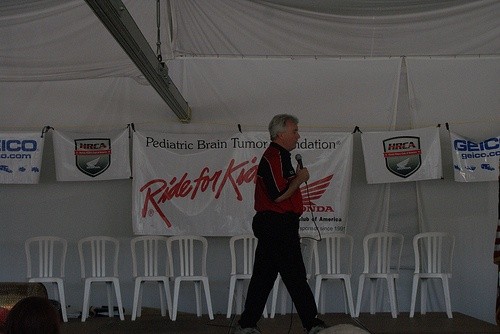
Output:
<box><xmin>235</xmin><ymin>114</ymin><xmax>330</xmax><ymax>334</ymax></box>
<box><xmin>2</xmin><ymin>296</ymin><xmax>62</xmax><ymax>334</ymax></box>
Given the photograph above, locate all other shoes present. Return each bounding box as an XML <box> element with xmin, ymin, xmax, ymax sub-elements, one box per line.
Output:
<box><xmin>303</xmin><ymin>324</ymin><xmax>332</xmax><ymax>334</ymax></box>
<box><xmin>238</xmin><ymin>326</ymin><xmax>263</xmax><ymax>334</ymax></box>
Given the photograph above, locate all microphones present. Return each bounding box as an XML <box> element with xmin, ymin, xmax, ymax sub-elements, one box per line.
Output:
<box><xmin>295</xmin><ymin>154</ymin><xmax>307</xmax><ymax>184</ymax></box>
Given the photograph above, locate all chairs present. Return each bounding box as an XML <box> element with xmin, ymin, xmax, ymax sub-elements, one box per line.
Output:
<box><xmin>270</xmin><ymin>231</ymin><xmax>314</xmax><ymax>319</ymax></box>
<box><xmin>315</xmin><ymin>233</ymin><xmax>355</xmax><ymax>316</ymax></box>
<box><xmin>78</xmin><ymin>236</ymin><xmax>125</xmax><ymax>321</ymax></box>
<box><xmin>226</xmin><ymin>234</ymin><xmax>267</xmax><ymax>319</ymax></box>
<box><xmin>129</xmin><ymin>236</ymin><xmax>173</xmax><ymax>319</ymax></box>
<box><xmin>166</xmin><ymin>236</ymin><xmax>215</xmax><ymax>320</ymax></box>
<box><xmin>409</xmin><ymin>232</ymin><xmax>455</xmax><ymax>317</ymax></box>
<box><xmin>356</xmin><ymin>232</ymin><xmax>405</xmax><ymax>318</ymax></box>
<box><xmin>25</xmin><ymin>236</ymin><xmax>69</xmax><ymax>323</ymax></box>
